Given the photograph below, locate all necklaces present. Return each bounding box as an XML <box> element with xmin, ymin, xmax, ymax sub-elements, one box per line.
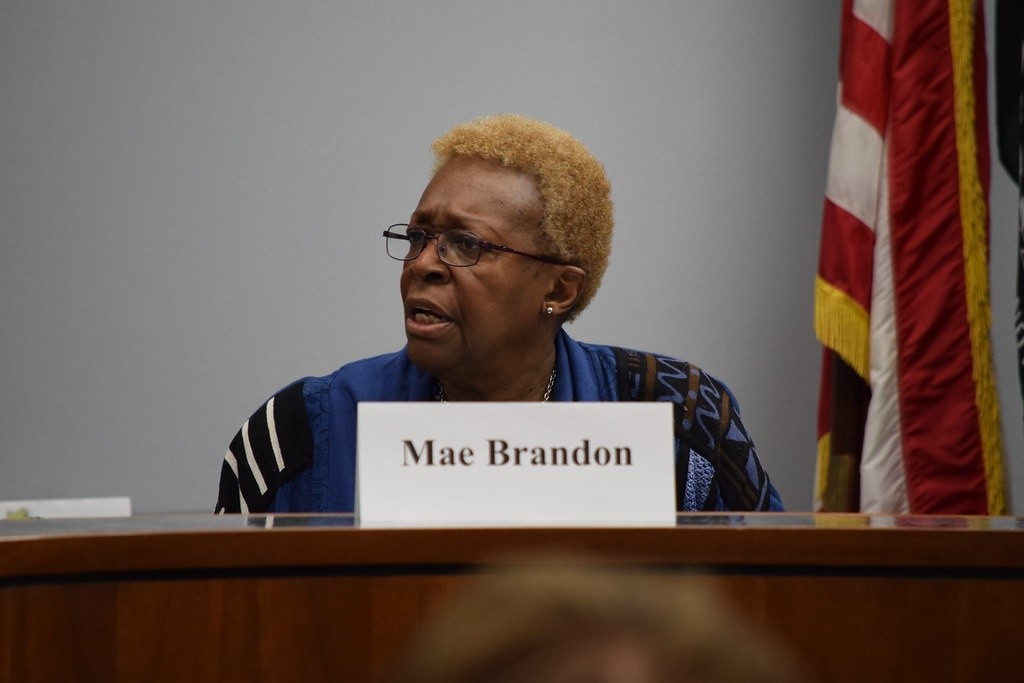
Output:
<box><xmin>437</xmin><ymin>360</ymin><xmax>558</xmax><ymax>401</ymax></box>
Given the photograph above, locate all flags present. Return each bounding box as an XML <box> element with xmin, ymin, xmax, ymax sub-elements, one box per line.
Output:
<box><xmin>813</xmin><ymin>0</ymin><xmax>1013</xmax><ymax>516</ymax></box>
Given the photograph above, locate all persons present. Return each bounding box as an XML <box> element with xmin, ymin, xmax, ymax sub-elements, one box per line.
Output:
<box><xmin>212</xmin><ymin>112</ymin><xmax>788</xmax><ymax>514</ymax></box>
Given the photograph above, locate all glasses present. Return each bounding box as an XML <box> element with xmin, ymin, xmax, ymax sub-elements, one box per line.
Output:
<box><xmin>383</xmin><ymin>224</ymin><xmax>568</xmax><ymax>267</ymax></box>
